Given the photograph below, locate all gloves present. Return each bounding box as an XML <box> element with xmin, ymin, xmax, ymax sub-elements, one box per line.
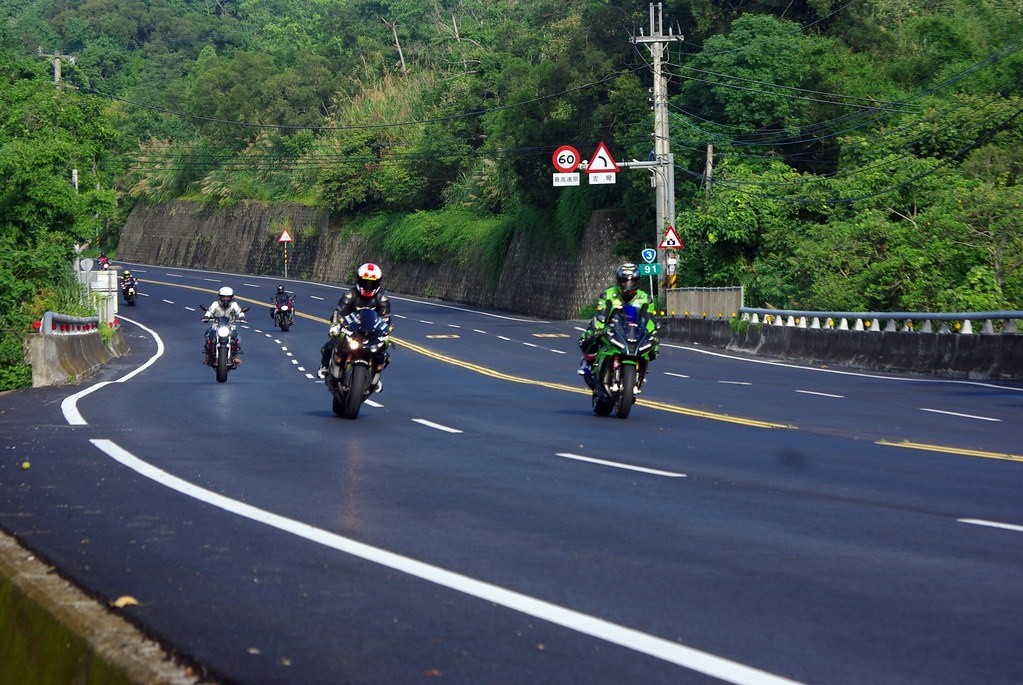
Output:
<box><xmin>203</xmin><ymin>315</ymin><xmax>209</xmax><ymax>323</ymax></box>
<box><xmin>329</xmin><ymin>323</ymin><xmax>341</xmax><ymax>336</ymax></box>
<box><xmin>239</xmin><ymin>317</ymin><xmax>246</xmax><ymax>324</ymax></box>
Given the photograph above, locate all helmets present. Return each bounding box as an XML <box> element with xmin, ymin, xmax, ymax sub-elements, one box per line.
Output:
<box><xmin>278</xmin><ymin>285</ymin><xmax>284</xmax><ymax>293</ymax></box>
<box><xmin>616</xmin><ymin>263</ymin><xmax>641</xmax><ymax>292</ymax></box>
<box><xmin>355</xmin><ymin>263</ymin><xmax>383</xmax><ymax>297</ymax></box>
<box><xmin>101</xmin><ymin>251</ymin><xmax>107</xmax><ymax>257</ymax></box>
<box><xmin>217</xmin><ymin>287</ymin><xmax>235</xmax><ymax>308</ymax></box>
<box><xmin>123</xmin><ymin>270</ymin><xmax>130</xmax><ymax>279</ymax></box>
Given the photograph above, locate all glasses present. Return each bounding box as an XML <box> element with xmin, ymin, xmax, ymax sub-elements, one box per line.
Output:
<box><xmin>620</xmin><ymin>275</ymin><xmax>639</xmax><ymax>284</ymax></box>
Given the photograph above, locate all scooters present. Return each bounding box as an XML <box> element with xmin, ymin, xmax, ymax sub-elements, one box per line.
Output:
<box><xmin>98</xmin><ymin>262</ymin><xmax>109</xmax><ymax>271</ymax></box>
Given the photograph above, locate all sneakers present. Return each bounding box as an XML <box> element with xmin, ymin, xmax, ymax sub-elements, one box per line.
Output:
<box><xmin>577</xmin><ymin>358</ymin><xmax>596</xmax><ymax>374</ymax></box>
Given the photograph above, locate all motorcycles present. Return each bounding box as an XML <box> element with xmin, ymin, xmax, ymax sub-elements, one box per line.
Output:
<box><xmin>198</xmin><ymin>303</ymin><xmax>250</xmax><ymax>383</ymax></box>
<box><xmin>321</xmin><ymin>306</ymin><xmax>398</xmax><ymax>419</ymax></box>
<box><xmin>577</xmin><ymin>293</ymin><xmax>669</xmax><ymax>420</ymax></box>
<box><xmin>272</xmin><ymin>297</ymin><xmax>295</xmax><ymax>333</ymax></box>
<box><xmin>119</xmin><ymin>277</ymin><xmax>139</xmax><ymax>307</ymax></box>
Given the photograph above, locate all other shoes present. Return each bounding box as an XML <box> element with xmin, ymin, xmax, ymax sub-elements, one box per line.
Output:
<box><xmin>316</xmin><ymin>364</ymin><xmax>329</xmax><ymax>379</ymax></box>
<box><xmin>374</xmin><ymin>379</ymin><xmax>383</xmax><ymax>393</ymax></box>
<box><xmin>205</xmin><ymin>356</ymin><xmax>211</xmax><ymax>364</ymax></box>
<box><xmin>233</xmin><ymin>357</ymin><xmax>240</xmax><ymax>364</ymax></box>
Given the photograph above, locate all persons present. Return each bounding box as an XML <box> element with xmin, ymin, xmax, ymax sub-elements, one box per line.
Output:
<box><xmin>120</xmin><ymin>270</ymin><xmax>138</xmax><ymax>300</ymax></box>
<box><xmin>317</xmin><ymin>263</ymin><xmax>391</xmax><ymax>393</ymax></box>
<box><xmin>97</xmin><ymin>251</ymin><xmax>111</xmax><ymax>267</ymax></box>
<box><xmin>201</xmin><ymin>287</ymin><xmax>245</xmax><ymax>366</ymax></box>
<box><xmin>577</xmin><ymin>264</ymin><xmax>660</xmax><ymax>374</ymax></box>
<box><xmin>272</xmin><ymin>285</ymin><xmax>295</xmax><ymax>327</ymax></box>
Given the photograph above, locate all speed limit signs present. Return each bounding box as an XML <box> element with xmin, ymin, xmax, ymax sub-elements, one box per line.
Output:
<box><xmin>551</xmin><ymin>145</ymin><xmax>580</xmax><ymax>173</ymax></box>
